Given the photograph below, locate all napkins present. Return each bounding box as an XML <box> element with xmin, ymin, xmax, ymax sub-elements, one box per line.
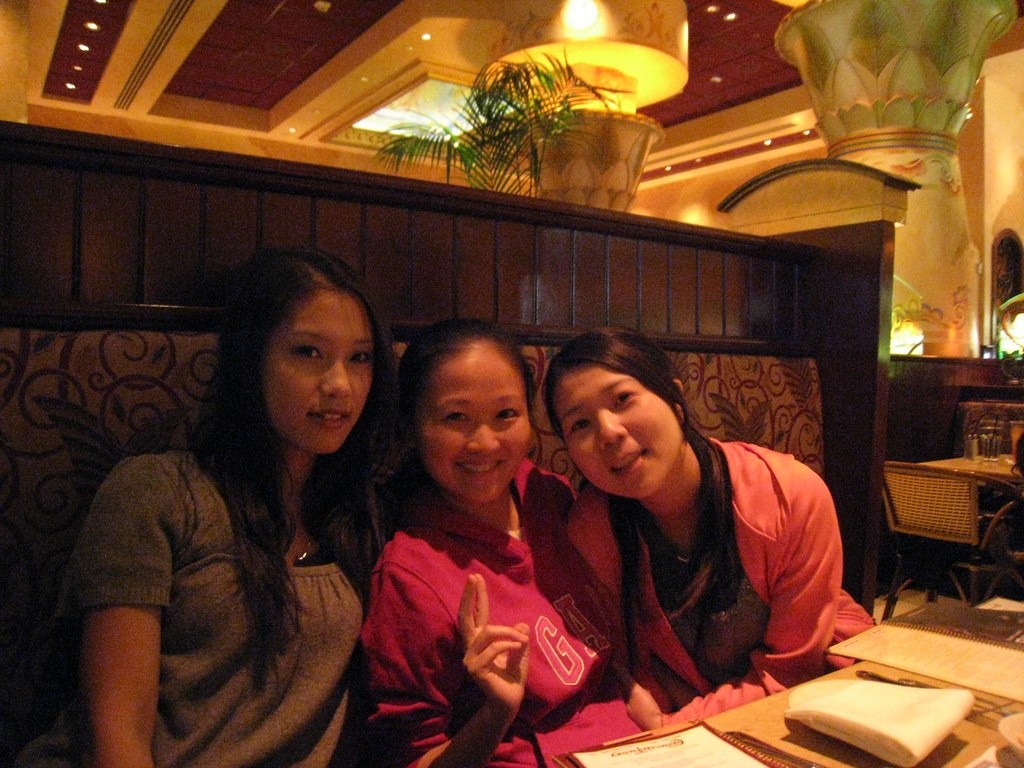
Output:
<box><xmin>785</xmin><ymin>680</ymin><xmax>975</xmax><ymax>768</ymax></box>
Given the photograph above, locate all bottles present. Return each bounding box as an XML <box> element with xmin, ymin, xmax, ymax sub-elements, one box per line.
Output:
<box><xmin>966</xmin><ymin>434</ymin><xmax>979</xmax><ymax>459</ymax></box>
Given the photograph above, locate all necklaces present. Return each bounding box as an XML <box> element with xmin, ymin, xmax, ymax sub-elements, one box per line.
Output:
<box><xmin>299</xmin><ymin>539</ymin><xmax>314</xmax><ymax>563</ymax></box>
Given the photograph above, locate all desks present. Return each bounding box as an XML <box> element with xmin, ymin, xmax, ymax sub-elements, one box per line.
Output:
<box><xmin>650</xmin><ymin>596</ymin><xmax>1022</xmax><ymax>768</ymax></box>
<box><xmin>916</xmin><ymin>452</ymin><xmax>1024</xmax><ymax>605</ymax></box>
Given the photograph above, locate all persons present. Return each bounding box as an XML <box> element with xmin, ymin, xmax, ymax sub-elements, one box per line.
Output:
<box><xmin>542</xmin><ymin>326</ymin><xmax>878</xmax><ymax>730</ymax></box>
<box><xmin>11</xmin><ymin>242</ymin><xmax>394</xmax><ymax>768</ymax></box>
<box><xmin>360</xmin><ymin>318</ymin><xmax>642</xmax><ymax>768</ymax></box>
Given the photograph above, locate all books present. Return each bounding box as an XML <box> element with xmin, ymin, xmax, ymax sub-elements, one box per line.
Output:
<box><xmin>550</xmin><ymin>616</ymin><xmax>1024</xmax><ymax>768</ymax></box>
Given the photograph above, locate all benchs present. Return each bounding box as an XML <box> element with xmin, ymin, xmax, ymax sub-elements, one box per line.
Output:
<box><xmin>0</xmin><ymin>301</ymin><xmax>826</xmax><ymax>768</ymax></box>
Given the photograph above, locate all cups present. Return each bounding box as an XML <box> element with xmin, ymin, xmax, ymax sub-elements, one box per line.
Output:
<box><xmin>981</xmin><ymin>420</ymin><xmax>1004</xmax><ymax>461</ymax></box>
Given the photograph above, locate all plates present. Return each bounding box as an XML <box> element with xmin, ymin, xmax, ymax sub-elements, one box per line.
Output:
<box><xmin>999</xmin><ymin>713</ymin><xmax>1024</xmax><ymax>759</ymax></box>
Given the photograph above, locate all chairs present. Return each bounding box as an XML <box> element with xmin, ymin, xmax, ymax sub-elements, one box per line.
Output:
<box><xmin>880</xmin><ymin>460</ymin><xmax>1024</xmax><ymax>622</ymax></box>
